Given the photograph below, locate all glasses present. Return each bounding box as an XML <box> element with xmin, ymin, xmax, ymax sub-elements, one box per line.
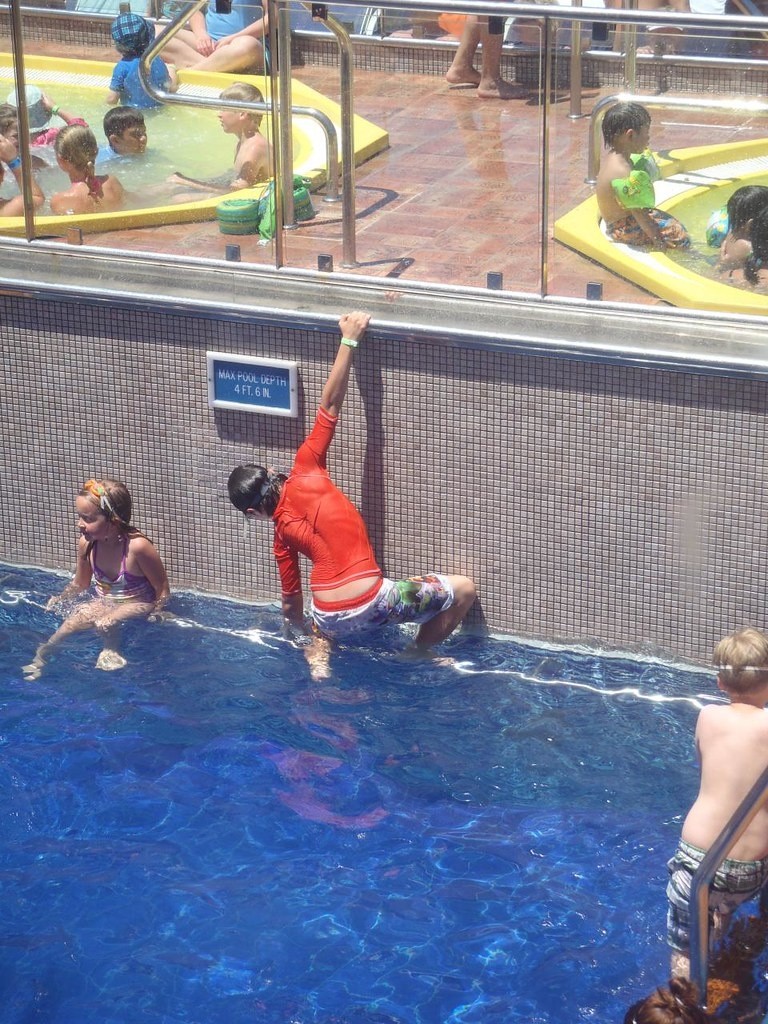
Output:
<box><xmin>85</xmin><ymin>479</ymin><xmax>105</xmax><ymax>496</ymax></box>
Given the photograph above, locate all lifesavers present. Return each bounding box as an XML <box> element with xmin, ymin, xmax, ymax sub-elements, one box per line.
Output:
<box><xmin>707</xmin><ymin>201</ymin><xmax>731</xmax><ymax>249</ymax></box>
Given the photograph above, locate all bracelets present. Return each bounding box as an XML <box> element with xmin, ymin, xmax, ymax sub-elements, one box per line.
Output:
<box><xmin>341</xmin><ymin>338</ymin><xmax>359</xmax><ymax>347</ymax></box>
<box><xmin>52</xmin><ymin>105</ymin><xmax>60</xmax><ymax>115</ymax></box>
<box><xmin>8</xmin><ymin>154</ymin><xmax>21</xmax><ymax>170</ymax></box>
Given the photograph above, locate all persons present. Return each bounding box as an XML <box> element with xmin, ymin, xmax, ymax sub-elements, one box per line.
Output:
<box><xmin>720</xmin><ymin>185</ymin><xmax>768</xmax><ymax>288</ymax></box>
<box><xmin>229</xmin><ymin>311</ymin><xmax>474</xmax><ymax>645</ymax></box>
<box><xmin>0</xmin><ymin>0</ymin><xmax>277</xmax><ymax>215</ymax></box>
<box><xmin>446</xmin><ymin>13</ymin><xmax>532</xmax><ymax>99</ymax></box>
<box><xmin>604</xmin><ymin>0</ymin><xmax>693</xmax><ymax>54</ymax></box>
<box><xmin>76</xmin><ymin>478</ymin><xmax>171</xmax><ymax>590</ymax></box>
<box><xmin>624</xmin><ymin>628</ymin><xmax>768</xmax><ymax>1024</ymax></box>
<box><xmin>596</xmin><ymin>103</ymin><xmax>689</xmax><ymax>245</ymax></box>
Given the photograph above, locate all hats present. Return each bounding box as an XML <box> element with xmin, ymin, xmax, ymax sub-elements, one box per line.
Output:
<box><xmin>7</xmin><ymin>86</ymin><xmax>53</xmax><ymax>130</ymax></box>
<box><xmin>112</xmin><ymin>12</ymin><xmax>155</xmax><ymax>59</ymax></box>
